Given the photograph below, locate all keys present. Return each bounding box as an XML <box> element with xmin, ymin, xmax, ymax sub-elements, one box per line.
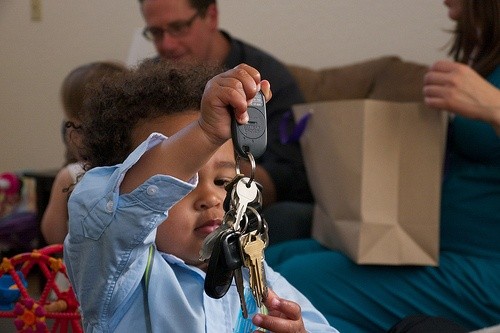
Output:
<box><xmin>202</xmin><ymin>91</ymin><xmax>268</xmax><ymax>319</ymax></box>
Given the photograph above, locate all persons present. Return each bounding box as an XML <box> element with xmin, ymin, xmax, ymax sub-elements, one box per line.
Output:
<box><xmin>137</xmin><ymin>0</ymin><xmax>316</xmax><ymax>245</ymax></box>
<box><xmin>64</xmin><ymin>53</ymin><xmax>338</xmax><ymax>333</ymax></box>
<box><xmin>41</xmin><ymin>60</ymin><xmax>129</xmax><ymax>244</ymax></box>
<box><xmin>265</xmin><ymin>0</ymin><xmax>500</xmax><ymax>333</ymax></box>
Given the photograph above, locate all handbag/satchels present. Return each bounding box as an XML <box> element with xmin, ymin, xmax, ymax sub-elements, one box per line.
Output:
<box><xmin>279</xmin><ymin>99</ymin><xmax>446</xmax><ymax>267</ymax></box>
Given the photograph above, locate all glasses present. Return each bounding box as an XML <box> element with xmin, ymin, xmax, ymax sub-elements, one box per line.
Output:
<box><xmin>142</xmin><ymin>10</ymin><xmax>201</xmax><ymax>42</ymax></box>
